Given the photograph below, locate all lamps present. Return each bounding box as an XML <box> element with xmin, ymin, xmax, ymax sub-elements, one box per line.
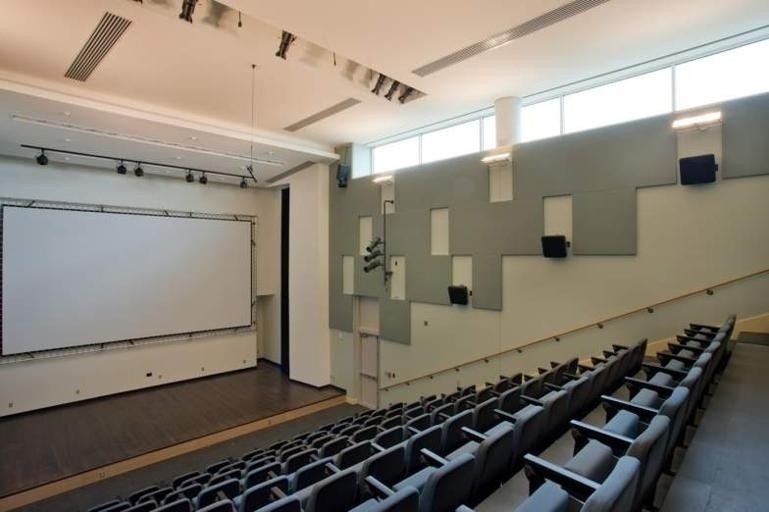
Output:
<box><xmin>364</xmin><ymin>248</ymin><xmax>384</xmax><ymax>262</ymax></box>
<box><xmin>364</xmin><ymin>259</ymin><xmax>383</xmax><ymax>273</ymax></box>
<box><xmin>384</xmin><ymin>80</ymin><xmax>400</xmax><ymax>101</ymax></box>
<box><xmin>366</xmin><ymin>236</ymin><xmax>383</xmax><ymax>251</ymax></box>
<box><xmin>21</xmin><ymin>143</ymin><xmax>257</xmax><ymax>189</ymax></box>
<box><xmin>399</xmin><ymin>86</ymin><xmax>414</xmax><ymax>104</ymax></box>
<box><xmin>371</xmin><ymin>73</ymin><xmax>386</xmax><ymax>95</ymax></box>
<box><xmin>179</xmin><ymin>0</ymin><xmax>198</xmax><ymax>23</ymax></box>
<box><xmin>276</xmin><ymin>30</ymin><xmax>293</xmax><ymax>59</ymax></box>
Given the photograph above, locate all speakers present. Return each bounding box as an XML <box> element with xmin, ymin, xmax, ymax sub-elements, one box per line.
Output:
<box><xmin>336</xmin><ymin>164</ymin><xmax>349</xmax><ymax>188</ymax></box>
<box><xmin>448</xmin><ymin>286</ymin><xmax>468</xmax><ymax>304</ymax></box>
<box><xmin>679</xmin><ymin>154</ymin><xmax>715</xmax><ymax>185</ymax></box>
<box><xmin>541</xmin><ymin>236</ymin><xmax>566</xmax><ymax>258</ymax></box>
